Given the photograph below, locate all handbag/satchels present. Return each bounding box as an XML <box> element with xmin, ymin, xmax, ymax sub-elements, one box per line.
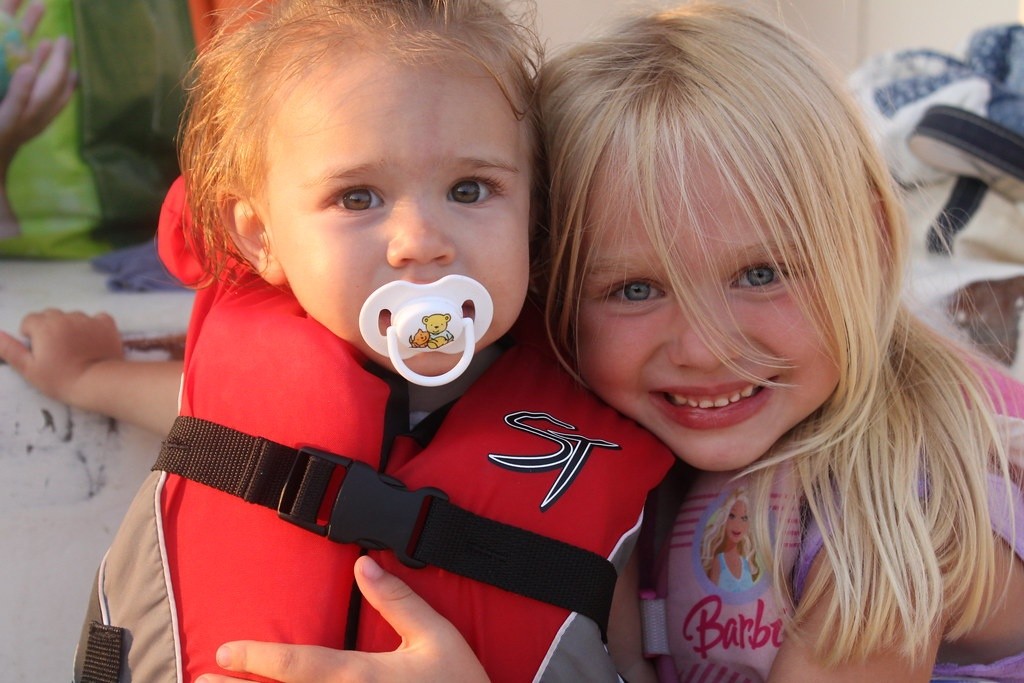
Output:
<box><xmin>0</xmin><ymin>0</ymin><xmax>198</xmax><ymax>260</ymax></box>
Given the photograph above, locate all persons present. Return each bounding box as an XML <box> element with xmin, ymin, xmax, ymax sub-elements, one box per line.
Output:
<box><xmin>0</xmin><ymin>0</ymin><xmax>1024</xmax><ymax>683</ymax></box>
<box><xmin>76</xmin><ymin>0</ymin><xmax>676</xmax><ymax>683</ymax></box>
